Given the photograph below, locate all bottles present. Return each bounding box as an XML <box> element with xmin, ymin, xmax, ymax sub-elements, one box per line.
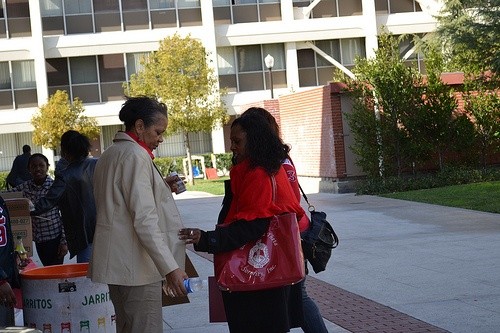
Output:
<box><xmin>16</xmin><ymin>236</ymin><xmax>26</xmax><ymax>258</ymax></box>
<box><xmin>170</xmin><ymin>171</ymin><xmax>186</xmax><ymax>194</ymax></box>
<box><xmin>163</xmin><ymin>277</ymin><xmax>208</xmax><ymax>297</ymax></box>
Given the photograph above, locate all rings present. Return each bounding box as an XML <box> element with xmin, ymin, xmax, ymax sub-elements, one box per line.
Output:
<box><xmin>190</xmin><ymin>230</ymin><xmax>193</xmax><ymax>235</ymax></box>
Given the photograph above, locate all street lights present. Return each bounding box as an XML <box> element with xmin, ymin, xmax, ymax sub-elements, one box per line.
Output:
<box><xmin>264</xmin><ymin>54</ymin><xmax>275</xmax><ymax>98</ymax></box>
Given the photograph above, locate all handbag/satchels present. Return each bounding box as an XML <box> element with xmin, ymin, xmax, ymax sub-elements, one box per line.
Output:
<box><xmin>300</xmin><ymin>205</ymin><xmax>339</xmax><ymax>273</ymax></box>
<box><xmin>214</xmin><ymin>164</ymin><xmax>305</xmax><ymax>292</ymax></box>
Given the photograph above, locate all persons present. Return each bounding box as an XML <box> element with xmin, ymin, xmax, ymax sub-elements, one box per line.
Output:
<box><xmin>0</xmin><ymin>152</ymin><xmax>68</xmax><ymax>268</ymax></box>
<box><xmin>242</xmin><ymin>108</ymin><xmax>331</xmax><ymax>333</ymax></box>
<box><xmin>6</xmin><ymin>145</ymin><xmax>32</xmax><ymax>190</ymax></box>
<box><xmin>178</xmin><ymin>116</ymin><xmax>306</xmax><ymax>333</ymax></box>
<box><xmin>87</xmin><ymin>94</ymin><xmax>190</xmax><ymax>333</ymax></box>
<box><xmin>0</xmin><ymin>197</ymin><xmax>24</xmax><ymax>333</ymax></box>
<box><xmin>32</xmin><ymin>130</ymin><xmax>98</xmax><ymax>264</ymax></box>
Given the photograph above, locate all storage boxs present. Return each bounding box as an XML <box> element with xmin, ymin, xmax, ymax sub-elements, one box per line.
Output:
<box><xmin>0</xmin><ymin>192</ymin><xmax>33</xmax><ymax>247</ymax></box>
